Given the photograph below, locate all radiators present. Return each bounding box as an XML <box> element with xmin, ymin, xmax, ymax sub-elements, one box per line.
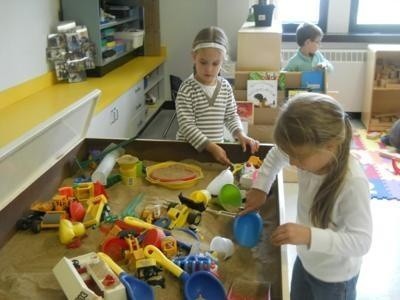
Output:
<box><xmin>281</xmin><ymin>48</ymin><xmax>366</xmax><ymax>113</ymax></box>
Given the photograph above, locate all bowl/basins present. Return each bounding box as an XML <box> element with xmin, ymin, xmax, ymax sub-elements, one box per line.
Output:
<box><xmin>209</xmin><ymin>165</ymin><xmax>233</xmax><ymax>196</ymax></box>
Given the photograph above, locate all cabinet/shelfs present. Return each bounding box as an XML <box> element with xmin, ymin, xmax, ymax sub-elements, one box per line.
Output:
<box><xmin>60</xmin><ymin>0</ymin><xmax>145</xmax><ymax>78</ymax></box>
<box><xmin>236</xmin><ymin>19</ymin><xmax>283</xmax><ymax>70</ymax></box>
<box><xmin>362</xmin><ymin>42</ymin><xmax>400</xmax><ymax>132</ymax></box>
<box><xmin>85</xmin><ymin>60</ymin><xmax>167</xmax><ymax>141</ymax></box>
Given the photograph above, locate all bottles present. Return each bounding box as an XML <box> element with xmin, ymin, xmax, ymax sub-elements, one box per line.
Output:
<box><xmin>92</xmin><ymin>147</ymin><xmax>120</xmax><ymax>184</ymax></box>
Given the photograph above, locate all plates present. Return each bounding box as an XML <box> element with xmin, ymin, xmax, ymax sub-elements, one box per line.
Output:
<box><xmin>146</xmin><ymin>161</ymin><xmax>203</xmax><ymax>189</ymax></box>
<box><xmin>150</xmin><ymin>167</ymin><xmax>198</xmax><ymax>181</ymax></box>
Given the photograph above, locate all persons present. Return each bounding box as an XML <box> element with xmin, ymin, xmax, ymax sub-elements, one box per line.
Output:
<box><xmin>175</xmin><ymin>26</ymin><xmax>260</xmax><ymax>166</ymax></box>
<box><xmin>280</xmin><ymin>22</ymin><xmax>334</xmax><ymax>72</ymax></box>
<box><xmin>238</xmin><ymin>92</ymin><xmax>373</xmax><ymax>300</ymax></box>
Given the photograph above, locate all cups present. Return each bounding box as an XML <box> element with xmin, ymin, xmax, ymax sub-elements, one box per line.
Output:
<box><xmin>114</xmin><ymin>155</ymin><xmax>138</xmax><ymax>186</ymax></box>
<box><xmin>210</xmin><ymin>236</ymin><xmax>235</xmax><ymax>260</ymax></box>
